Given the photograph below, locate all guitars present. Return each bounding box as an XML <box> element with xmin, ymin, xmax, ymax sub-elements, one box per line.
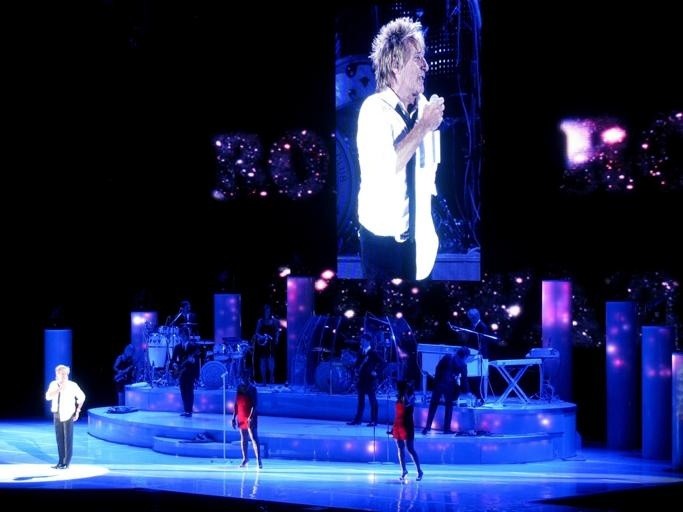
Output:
<box><xmin>171</xmin><ymin>346</ymin><xmax>202</xmax><ymax>380</ymax></box>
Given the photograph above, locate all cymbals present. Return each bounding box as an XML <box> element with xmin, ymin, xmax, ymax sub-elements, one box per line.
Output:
<box><xmin>181</xmin><ymin>323</ymin><xmax>198</xmax><ymax>327</ymax></box>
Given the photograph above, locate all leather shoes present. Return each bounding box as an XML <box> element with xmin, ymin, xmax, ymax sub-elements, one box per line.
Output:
<box><xmin>59</xmin><ymin>465</ymin><xmax>68</xmax><ymax>469</ymax></box>
<box><xmin>51</xmin><ymin>462</ymin><xmax>64</xmax><ymax>469</ymax></box>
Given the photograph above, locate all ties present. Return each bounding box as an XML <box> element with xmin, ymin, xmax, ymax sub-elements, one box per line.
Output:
<box><xmin>397</xmin><ymin>104</ymin><xmax>418</xmax><ymax>245</ymax></box>
<box><xmin>183</xmin><ymin>343</ymin><xmax>186</xmax><ymax>350</ymax></box>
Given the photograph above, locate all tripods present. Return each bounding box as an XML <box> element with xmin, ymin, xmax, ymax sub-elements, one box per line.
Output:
<box><xmin>135</xmin><ymin>322</ymin><xmax>178</xmax><ymax>388</ymax></box>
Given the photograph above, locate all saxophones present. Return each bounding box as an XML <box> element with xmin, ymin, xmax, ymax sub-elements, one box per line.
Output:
<box><xmin>114</xmin><ymin>364</ymin><xmax>137</xmax><ymax>382</ymax></box>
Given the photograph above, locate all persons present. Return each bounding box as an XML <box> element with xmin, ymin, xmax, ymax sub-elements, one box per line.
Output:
<box><xmin>386</xmin><ymin>380</ymin><xmax>424</xmax><ymax>480</ymax></box>
<box><xmin>346</xmin><ymin>333</ymin><xmax>383</xmax><ymax>427</ymax></box>
<box><xmin>45</xmin><ymin>365</ymin><xmax>87</xmax><ymax>469</ymax></box>
<box><xmin>114</xmin><ymin>299</ymin><xmax>202</xmax><ymax>417</ymax></box>
<box><xmin>352</xmin><ymin>16</ymin><xmax>448</xmax><ymax>282</ymax></box>
<box><xmin>256</xmin><ymin>305</ymin><xmax>278</xmax><ymax>385</ymax></box>
<box><xmin>419</xmin><ymin>347</ymin><xmax>471</xmax><ymax>436</ymax></box>
<box><xmin>230</xmin><ymin>370</ymin><xmax>264</xmax><ymax>471</ymax></box>
<box><xmin>467</xmin><ymin>308</ymin><xmax>487</xmax><ymax>408</ymax></box>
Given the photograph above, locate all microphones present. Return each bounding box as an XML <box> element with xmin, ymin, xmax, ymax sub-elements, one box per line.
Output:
<box><xmin>448</xmin><ymin>321</ymin><xmax>452</xmax><ymax>328</ymax></box>
<box><xmin>430</xmin><ymin>94</ymin><xmax>441</xmax><ymax>164</ymax></box>
<box><xmin>386</xmin><ymin>431</ymin><xmax>392</xmax><ymax>434</ymax></box>
<box><xmin>220</xmin><ymin>371</ymin><xmax>228</xmax><ymax>378</ymax></box>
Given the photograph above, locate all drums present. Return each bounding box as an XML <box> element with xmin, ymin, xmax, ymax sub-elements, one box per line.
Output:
<box><xmin>168</xmin><ymin>334</ymin><xmax>182</xmax><ymax>363</ymax></box>
<box><xmin>201</xmin><ymin>361</ymin><xmax>227</xmax><ymax>389</ymax></box>
<box><xmin>158</xmin><ymin>327</ymin><xmax>179</xmax><ymax>335</ymax></box>
<box><xmin>229</xmin><ymin>343</ymin><xmax>244</xmax><ymax>359</ymax></box>
<box><xmin>149</xmin><ymin>333</ymin><xmax>167</xmax><ymax>368</ymax></box>
<box><xmin>213</xmin><ymin>343</ymin><xmax>229</xmax><ymax>361</ymax></box>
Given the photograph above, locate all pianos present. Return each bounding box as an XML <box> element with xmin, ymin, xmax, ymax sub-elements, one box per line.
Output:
<box><xmin>418</xmin><ymin>344</ymin><xmax>488</xmax><ymax>402</ymax></box>
<box><xmin>489</xmin><ymin>359</ymin><xmax>542</xmax><ymax>366</ymax></box>
